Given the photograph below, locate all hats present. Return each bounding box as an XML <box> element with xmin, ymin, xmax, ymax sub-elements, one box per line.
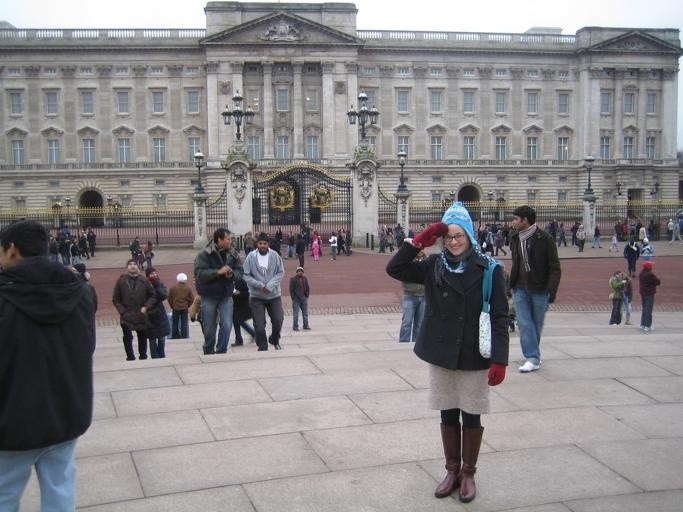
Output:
<box><xmin>643</xmin><ymin>238</ymin><xmax>648</xmax><ymax>242</ymax></box>
<box><xmin>296</xmin><ymin>266</ymin><xmax>304</xmax><ymax>272</ymax></box>
<box><xmin>128</xmin><ymin>259</ymin><xmax>138</xmax><ymax>266</ymax></box>
<box><xmin>257</xmin><ymin>232</ymin><xmax>270</xmax><ymax>243</ymax></box>
<box><xmin>630</xmin><ymin>240</ymin><xmax>634</xmax><ymax>245</ymax></box>
<box><xmin>145</xmin><ymin>267</ymin><xmax>155</xmax><ymax>276</ymax></box>
<box><xmin>440</xmin><ymin>202</ymin><xmax>498</xmax><ymax>273</ymax></box>
<box><xmin>641</xmin><ymin>261</ymin><xmax>656</xmax><ymax>267</ymax></box>
<box><xmin>74</xmin><ymin>262</ymin><xmax>86</xmax><ymax>272</ymax></box>
<box><xmin>177</xmin><ymin>273</ymin><xmax>188</xmax><ymax>280</ymax></box>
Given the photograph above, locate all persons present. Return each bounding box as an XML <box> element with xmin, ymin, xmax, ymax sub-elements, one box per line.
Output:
<box><xmin>639</xmin><ymin>261</ymin><xmax>661</xmax><ymax>332</ymax></box>
<box><xmin>623</xmin><ymin>240</ymin><xmax>640</xmax><ymax>278</ymax></box>
<box><xmin>137</xmin><ymin>250</ymin><xmax>145</xmax><ymax>270</ymax></box>
<box><xmin>639</xmin><ymin>238</ymin><xmax>654</xmax><ymax>261</ymax></box>
<box><xmin>648</xmin><ymin>220</ymin><xmax>657</xmax><ymax>234</ymax></box>
<box><xmin>295</xmin><ymin>233</ymin><xmax>305</xmax><ymax>270</ymax></box>
<box><xmin>621</xmin><ymin>220</ymin><xmax>628</xmax><ymax>236</ymax></box>
<box><xmin>378</xmin><ymin>226</ymin><xmax>386</xmax><ymax>254</ymax></box>
<box><xmin>338</xmin><ymin>228</ymin><xmax>346</xmax><ymax>255</ymax></box>
<box><xmin>667</xmin><ymin>219</ymin><xmax>674</xmax><ymax>242</ymax></box>
<box><xmin>245</xmin><ymin>232</ymin><xmax>256</xmax><ymax>255</ymax></box>
<box><xmin>393</xmin><ymin>223</ymin><xmax>404</xmax><ymax>232</ymax></box>
<box><xmin>609</xmin><ymin>270</ymin><xmax>626</xmax><ymax>325</ymax></box>
<box><xmin>576</xmin><ymin>225</ymin><xmax>586</xmax><ymax>253</ymax></box>
<box><xmin>287</xmin><ymin>231</ymin><xmax>294</xmax><ymax>258</ymax></box>
<box><xmin>407</xmin><ymin>229</ymin><xmax>415</xmax><ymax>238</ymax></box>
<box><xmin>615</xmin><ymin>220</ymin><xmax>623</xmax><ymax>242</ymax></box>
<box><xmin>129</xmin><ymin>236</ymin><xmax>140</xmax><ymax>260</ymax></box>
<box><xmin>243</xmin><ymin>235</ymin><xmax>285</xmax><ymax>351</ymax></box>
<box><xmin>545</xmin><ymin>222</ymin><xmax>552</xmax><ymax>233</ymax></box>
<box><xmin>63</xmin><ymin>225</ymin><xmax>69</xmax><ymax>235</ymax></box>
<box><xmin>669</xmin><ymin>220</ymin><xmax>683</xmax><ymax>246</ymax></box>
<box><xmin>71</xmin><ymin>240</ymin><xmax>79</xmax><ymax>265</ymax></box>
<box><xmin>168</xmin><ymin>273</ymin><xmax>194</xmax><ymax>339</ymax></box>
<box><xmin>275</xmin><ymin>227</ymin><xmax>282</xmax><ymax>242</ymax></box>
<box><xmin>494</xmin><ymin>225</ymin><xmax>507</xmax><ymax>256</ymax></box>
<box><xmin>144</xmin><ymin>241</ymin><xmax>154</xmax><ymax>269</ymax></box>
<box><xmin>387</xmin><ymin>231</ymin><xmax>395</xmax><ymax>253</ymax></box>
<box><xmin>345</xmin><ymin>229</ymin><xmax>353</xmax><ymax>256</ymax></box>
<box><xmin>289</xmin><ymin>266</ymin><xmax>312</xmax><ymax>331</ymax></box>
<box><xmin>50</xmin><ymin>238</ymin><xmax>61</xmax><ymax>261</ymax></box>
<box><xmin>243</xmin><ymin>233</ymin><xmax>248</xmax><ymax>249</ymax></box>
<box><xmin>193</xmin><ymin>228</ymin><xmax>243</xmax><ymax>355</ymax></box>
<box><xmin>591</xmin><ymin>224</ymin><xmax>603</xmax><ymax>249</ymax></box>
<box><xmin>636</xmin><ymin>220</ymin><xmax>642</xmax><ymax>235</ymax></box>
<box><xmin>630</xmin><ymin>221</ymin><xmax>635</xmax><ymax>235</ymax></box>
<box><xmin>551</xmin><ymin>218</ymin><xmax>559</xmax><ymax>239</ymax></box>
<box><xmin>79</xmin><ymin>231</ymin><xmax>89</xmax><ymax>261</ymax></box>
<box><xmin>112</xmin><ymin>259</ymin><xmax>157</xmax><ymax>360</ymax></box>
<box><xmin>386</xmin><ymin>201</ymin><xmax>509</xmax><ymax>502</ymax></box>
<box><xmin>143</xmin><ymin>268</ymin><xmax>171</xmax><ymax>359</ymax></box>
<box><xmin>620</xmin><ymin>272</ymin><xmax>633</xmax><ymax>327</ymax></box>
<box><xmin>397</xmin><ymin>231</ymin><xmax>405</xmax><ymax>245</ymax></box>
<box><xmin>0</xmin><ymin>220</ymin><xmax>98</xmax><ymax>512</ymax></box>
<box><xmin>558</xmin><ymin>223</ymin><xmax>567</xmax><ymax>247</ymax></box>
<box><xmin>328</xmin><ymin>232</ymin><xmax>338</xmax><ymax>261</ymax></box>
<box><xmin>59</xmin><ymin>235</ymin><xmax>69</xmax><ymax>265</ymax></box>
<box><xmin>312</xmin><ymin>231</ymin><xmax>321</xmax><ymax>263</ymax></box>
<box><xmin>571</xmin><ymin>221</ymin><xmax>579</xmax><ymax>246</ymax></box>
<box><xmin>476</xmin><ymin>222</ymin><xmax>495</xmax><ymax>256</ymax></box>
<box><xmin>509</xmin><ymin>206</ymin><xmax>562</xmax><ymax>372</ymax></box>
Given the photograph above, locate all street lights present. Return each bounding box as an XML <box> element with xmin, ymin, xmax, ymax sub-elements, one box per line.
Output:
<box><xmin>194</xmin><ymin>150</ymin><xmax>204</xmax><ymax>193</ymax></box>
<box><xmin>193</xmin><ymin>149</ymin><xmax>205</xmax><ymax>194</ymax></box>
<box><xmin>107</xmin><ymin>195</ymin><xmax>113</xmax><ymax>214</ymax></box>
<box><xmin>487</xmin><ymin>190</ymin><xmax>493</xmax><ymax>210</ymax></box>
<box><xmin>65</xmin><ymin>195</ymin><xmax>70</xmax><ymax>216</ymax></box>
<box><xmin>450</xmin><ymin>191</ymin><xmax>455</xmax><ymax>206</ymax></box>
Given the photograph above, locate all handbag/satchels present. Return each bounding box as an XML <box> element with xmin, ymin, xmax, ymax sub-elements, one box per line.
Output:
<box><xmin>476</xmin><ymin>261</ymin><xmax>506</xmax><ymax>359</ymax></box>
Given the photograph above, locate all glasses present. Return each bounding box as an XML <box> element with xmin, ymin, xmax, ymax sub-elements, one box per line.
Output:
<box><xmin>443</xmin><ymin>233</ymin><xmax>466</xmax><ymax>241</ymax></box>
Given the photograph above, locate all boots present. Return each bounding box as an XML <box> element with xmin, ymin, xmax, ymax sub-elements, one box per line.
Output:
<box><xmin>434</xmin><ymin>424</ymin><xmax>462</xmax><ymax>498</ymax></box>
<box><xmin>460</xmin><ymin>426</ymin><xmax>485</xmax><ymax>502</ymax></box>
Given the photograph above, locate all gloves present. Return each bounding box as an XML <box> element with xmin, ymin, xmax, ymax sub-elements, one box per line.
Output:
<box><xmin>506</xmin><ymin>286</ymin><xmax>514</xmax><ymax>297</ymax></box>
<box><xmin>414</xmin><ymin>221</ymin><xmax>447</xmax><ymax>248</ymax></box>
<box><xmin>622</xmin><ymin>280</ymin><xmax>627</xmax><ymax>283</ymax></box>
<box><xmin>546</xmin><ymin>289</ymin><xmax>555</xmax><ymax>304</ymax></box>
<box><xmin>486</xmin><ymin>365</ymin><xmax>507</xmax><ymax>386</ymax></box>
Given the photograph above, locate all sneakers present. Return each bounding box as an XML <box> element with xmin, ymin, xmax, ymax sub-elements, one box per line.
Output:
<box><xmin>271</xmin><ymin>343</ymin><xmax>281</xmax><ymax>350</ymax></box>
<box><xmin>293</xmin><ymin>328</ymin><xmax>299</xmax><ymax>331</ymax></box>
<box><xmin>231</xmin><ymin>343</ymin><xmax>244</xmax><ymax>347</ymax></box>
<box><xmin>624</xmin><ymin>321</ymin><xmax>633</xmax><ymax>325</ymax></box>
<box><xmin>518</xmin><ymin>361</ymin><xmax>541</xmax><ymax>372</ymax></box>
<box><xmin>191</xmin><ymin>317</ymin><xmax>195</xmax><ymax>322</ymax></box>
<box><xmin>639</xmin><ymin>325</ymin><xmax>645</xmax><ymax>329</ymax></box>
<box><xmin>644</xmin><ymin>327</ymin><xmax>655</xmax><ymax>333</ymax></box>
<box><xmin>302</xmin><ymin>327</ymin><xmax>311</xmax><ymax>330</ymax></box>
